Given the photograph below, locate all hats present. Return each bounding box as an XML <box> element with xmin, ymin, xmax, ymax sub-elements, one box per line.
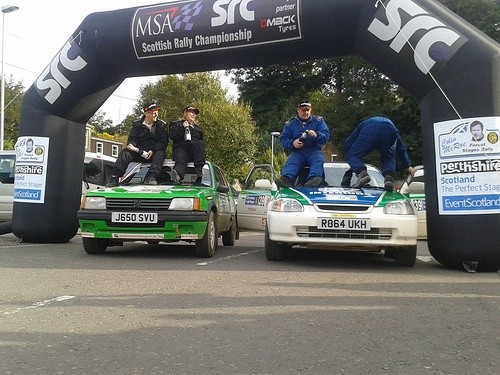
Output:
<box><xmin>143</xmin><ymin>102</ymin><xmax>161</xmax><ymax>112</ymax></box>
<box><xmin>183</xmin><ymin>106</ymin><xmax>199</xmax><ymax>114</ymax></box>
<box><xmin>297</xmin><ymin>100</ymin><xmax>311</xmax><ymax>108</ymax></box>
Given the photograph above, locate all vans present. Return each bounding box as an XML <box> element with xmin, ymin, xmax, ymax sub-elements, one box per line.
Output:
<box><xmin>0</xmin><ymin>150</ymin><xmax>119</xmax><ymax>221</ymax></box>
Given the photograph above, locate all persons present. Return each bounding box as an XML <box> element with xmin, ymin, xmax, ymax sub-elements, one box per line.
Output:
<box><xmin>342</xmin><ymin>114</ymin><xmax>415</xmax><ymax>192</ymax></box>
<box><xmin>468</xmin><ymin>120</ymin><xmax>485</xmax><ymax>143</ymax></box>
<box><xmin>274</xmin><ymin>99</ymin><xmax>331</xmax><ymax>188</ymax></box>
<box><xmin>26</xmin><ymin>139</ymin><xmax>34</xmax><ymax>153</ymax></box>
<box><xmin>105</xmin><ymin>102</ymin><xmax>169</xmax><ymax>188</ymax></box>
<box><xmin>167</xmin><ymin>105</ymin><xmax>207</xmax><ymax>184</ymax></box>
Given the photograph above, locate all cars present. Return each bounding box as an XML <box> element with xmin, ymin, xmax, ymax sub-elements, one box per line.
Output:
<box><xmin>78</xmin><ymin>159</ymin><xmax>239</xmax><ymax>257</ymax></box>
<box><xmin>237</xmin><ymin>162</ymin><xmax>425</xmax><ymax>265</ymax></box>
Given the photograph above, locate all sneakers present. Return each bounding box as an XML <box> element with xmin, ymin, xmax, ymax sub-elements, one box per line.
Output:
<box><xmin>384</xmin><ymin>175</ymin><xmax>393</xmax><ymax>190</ymax></box>
<box><xmin>352</xmin><ymin>171</ymin><xmax>371</xmax><ymax>188</ymax></box>
<box><xmin>304</xmin><ymin>177</ymin><xmax>322</xmax><ymax>187</ymax></box>
<box><xmin>170</xmin><ymin>170</ymin><xmax>180</xmax><ymax>185</ymax></box>
<box><xmin>193</xmin><ymin>176</ymin><xmax>205</xmax><ymax>186</ymax></box>
<box><xmin>275</xmin><ymin>177</ymin><xmax>290</xmax><ymax>188</ymax></box>
<box><xmin>144</xmin><ymin>174</ymin><xmax>157</xmax><ymax>185</ymax></box>
<box><xmin>106</xmin><ymin>175</ymin><xmax>119</xmax><ymax>187</ymax></box>
